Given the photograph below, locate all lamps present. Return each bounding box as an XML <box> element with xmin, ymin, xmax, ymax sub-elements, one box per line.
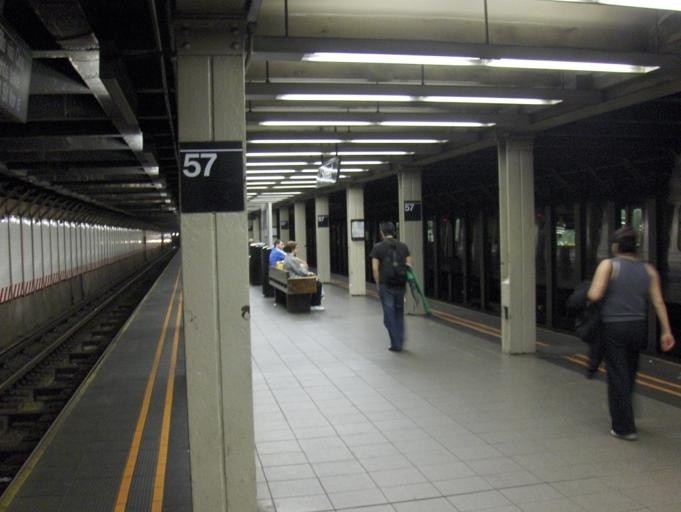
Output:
<box><xmin>246</xmin><ymin>0</ymin><xmax>661</xmax><ymax>213</ymax></box>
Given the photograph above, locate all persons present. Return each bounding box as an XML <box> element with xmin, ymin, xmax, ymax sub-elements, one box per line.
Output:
<box><xmin>584</xmin><ymin>225</ymin><xmax>676</xmax><ymax>440</ymax></box>
<box><xmin>281</xmin><ymin>240</ymin><xmax>325</xmax><ymax>311</ymax></box>
<box><xmin>367</xmin><ymin>220</ymin><xmax>411</xmax><ymax>352</ymax></box>
<box><xmin>267</xmin><ymin>238</ymin><xmax>285</xmax><ymax>267</ymax></box>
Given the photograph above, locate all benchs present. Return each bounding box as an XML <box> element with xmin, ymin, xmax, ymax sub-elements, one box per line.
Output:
<box><xmin>268</xmin><ymin>265</ymin><xmax>318</xmax><ymax>313</ymax></box>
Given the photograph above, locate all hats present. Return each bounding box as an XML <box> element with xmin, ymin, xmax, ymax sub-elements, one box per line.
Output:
<box><xmin>612</xmin><ymin>225</ymin><xmax>637</xmax><ymax>242</ymax></box>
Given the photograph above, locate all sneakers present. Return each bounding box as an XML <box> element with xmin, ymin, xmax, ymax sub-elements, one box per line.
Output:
<box><xmin>611</xmin><ymin>426</ymin><xmax>638</xmax><ymax>440</ymax></box>
<box><xmin>311</xmin><ymin>305</ymin><xmax>325</xmax><ymax>311</ymax></box>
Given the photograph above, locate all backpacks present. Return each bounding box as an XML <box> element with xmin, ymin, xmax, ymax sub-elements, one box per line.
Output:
<box><xmin>380</xmin><ymin>240</ymin><xmax>408</xmax><ymax>289</ymax></box>
